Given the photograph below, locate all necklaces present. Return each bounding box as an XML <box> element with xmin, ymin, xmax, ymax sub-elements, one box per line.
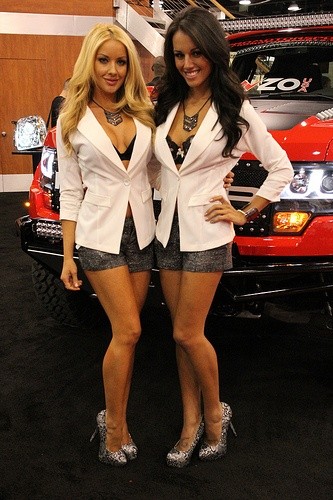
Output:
<box><xmin>181</xmin><ymin>96</ymin><xmax>211</xmax><ymax>132</ymax></box>
<box><xmin>90</xmin><ymin>98</ymin><xmax>123</xmax><ymax>126</ymax></box>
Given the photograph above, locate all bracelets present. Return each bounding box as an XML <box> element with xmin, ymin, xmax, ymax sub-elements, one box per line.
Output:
<box><xmin>238</xmin><ymin>202</ymin><xmax>260</xmax><ymax>222</ymax></box>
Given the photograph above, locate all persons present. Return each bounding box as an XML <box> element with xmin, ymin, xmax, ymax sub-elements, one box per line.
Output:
<box><xmin>55</xmin><ymin>23</ymin><xmax>234</xmax><ymax>466</ymax></box>
<box><xmin>51</xmin><ymin>79</ymin><xmax>72</xmax><ymax>127</ymax></box>
<box><xmin>151</xmin><ymin>7</ymin><xmax>294</xmax><ymax>467</ymax></box>
<box><xmin>146</xmin><ymin>56</ymin><xmax>165</xmax><ymax>86</ymax></box>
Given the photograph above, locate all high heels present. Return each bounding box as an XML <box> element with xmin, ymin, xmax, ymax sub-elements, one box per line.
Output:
<box><xmin>166</xmin><ymin>414</ymin><xmax>205</xmax><ymax>469</ymax></box>
<box><xmin>198</xmin><ymin>402</ymin><xmax>237</xmax><ymax>462</ymax></box>
<box><xmin>120</xmin><ymin>432</ymin><xmax>139</xmax><ymax>461</ymax></box>
<box><xmin>90</xmin><ymin>410</ymin><xmax>127</xmax><ymax>467</ymax></box>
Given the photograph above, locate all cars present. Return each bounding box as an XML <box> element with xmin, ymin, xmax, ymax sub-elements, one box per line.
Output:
<box><xmin>16</xmin><ymin>10</ymin><xmax>333</xmax><ymax>303</ymax></box>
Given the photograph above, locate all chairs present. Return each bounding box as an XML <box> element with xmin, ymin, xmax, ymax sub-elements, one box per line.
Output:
<box><xmin>261</xmin><ymin>53</ymin><xmax>322</xmax><ymax>94</ymax></box>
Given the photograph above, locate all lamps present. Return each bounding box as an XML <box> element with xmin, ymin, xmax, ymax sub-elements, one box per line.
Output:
<box><xmin>288</xmin><ymin>0</ymin><xmax>299</xmax><ymax>10</ymax></box>
